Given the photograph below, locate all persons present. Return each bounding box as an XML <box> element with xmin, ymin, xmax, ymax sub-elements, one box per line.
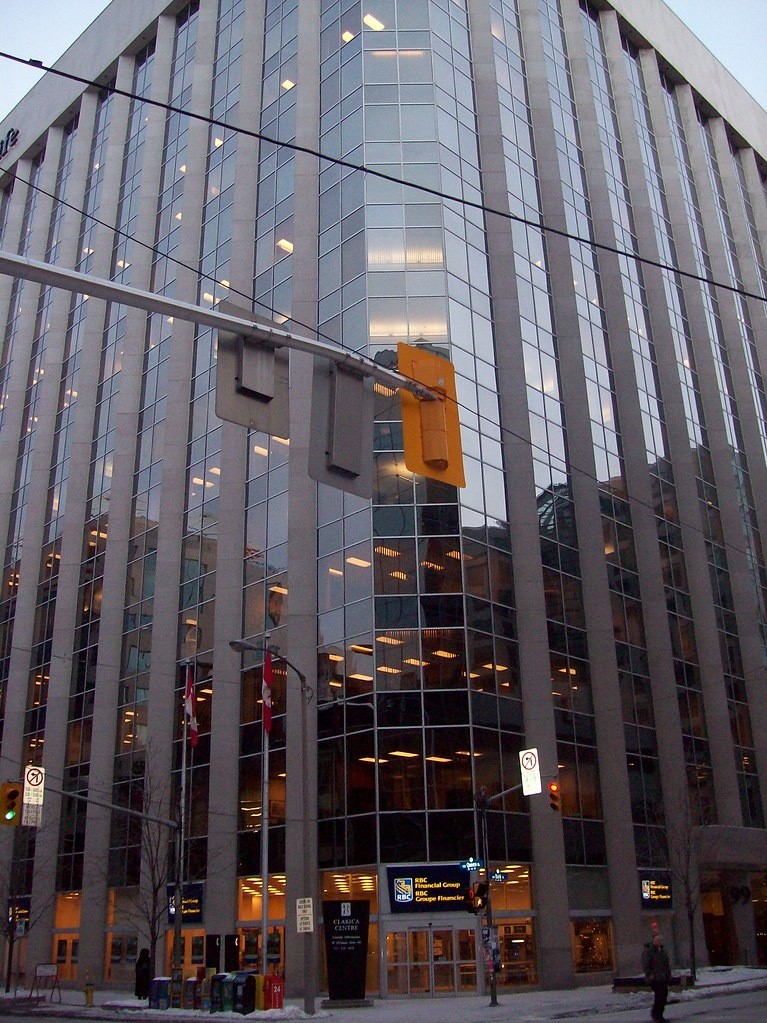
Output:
<box><xmin>134</xmin><ymin>948</ymin><xmax>150</xmax><ymax>1000</ymax></box>
<box><xmin>580</xmin><ymin>933</ymin><xmax>595</xmax><ymax>960</ymax></box>
<box><xmin>641</xmin><ymin>934</ymin><xmax>673</xmax><ymax>1023</ymax></box>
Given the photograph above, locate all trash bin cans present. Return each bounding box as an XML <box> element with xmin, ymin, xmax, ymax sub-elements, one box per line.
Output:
<box><xmin>150</xmin><ymin>967</ymin><xmax>284</xmax><ymax>1015</ymax></box>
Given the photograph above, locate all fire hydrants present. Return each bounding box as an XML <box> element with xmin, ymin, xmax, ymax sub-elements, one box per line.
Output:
<box><xmin>83</xmin><ymin>980</ymin><xmax>94</xmax><ymax>1006</ymax></box>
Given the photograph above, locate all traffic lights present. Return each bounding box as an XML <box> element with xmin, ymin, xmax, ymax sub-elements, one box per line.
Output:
<box><xmin>0</xmin><ymin>782</ymin><xmax>23</xmax><ymax>826</ymax></box>
<box><xmin>549</xmin><ymin>782</ymin><xmax>562</xmax><ymax>813</ymax></box>
<box><xmin>473</xmin><ymin>895</ymin><xmax>487</xmax><ymax>910</ymax></box>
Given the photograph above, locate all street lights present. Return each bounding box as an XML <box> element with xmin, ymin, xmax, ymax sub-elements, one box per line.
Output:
<box><xmin>228</xmin><ymin>639</ymin><xmax>315</xmax><ymax>1015</ymax></box>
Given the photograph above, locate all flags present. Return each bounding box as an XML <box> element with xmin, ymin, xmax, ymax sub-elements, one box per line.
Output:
<box><xmin>186</xmin><ymin>668</ymin><xmax>197</xmax><ymax>748</ymax></box>
<box><xmin>262</xmin><ymin>642</ymin><xmax>275</xmax><ymax>732</ymax></box>
